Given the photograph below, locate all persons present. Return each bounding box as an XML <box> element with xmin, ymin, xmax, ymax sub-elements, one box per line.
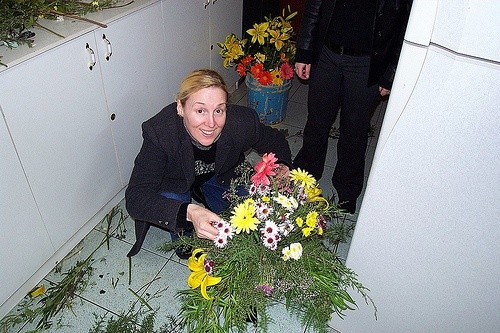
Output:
<box><xmin>292</xmin><ymin>0</ymin><xmax>413</xmax><ymax>216</ymax></box>
<box><xmin>125</xmin><ymin>69</ymin><xmax>292</xmax><ymax>260</ymax></box>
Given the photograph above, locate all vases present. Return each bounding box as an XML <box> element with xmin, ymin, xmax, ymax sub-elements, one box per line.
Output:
<box><xmin>244</xmin><ymin>74</ymin><xmax>292</xmax><ymax>125</ymax></box>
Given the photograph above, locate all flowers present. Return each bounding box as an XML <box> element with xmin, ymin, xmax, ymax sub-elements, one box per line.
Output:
<box><xmin>217</xmin><ymin>5</ymin><xmax>298</xmax><ymax>87</ymax></box>
<box><xmin>156</xmin><ymin>148</ymin><xmax>377</xmax><ymax>333</ymax></box>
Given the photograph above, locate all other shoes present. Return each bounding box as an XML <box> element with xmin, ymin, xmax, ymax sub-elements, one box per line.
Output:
<box><xmin>337</xmin><ymin>200</ymin><xmax>356</xmax><ymax>214</ymax></box>
<box><xmin>171</xmin><ymin>231</ymin><xmax>193</xmax><ymax>259</ymax></box>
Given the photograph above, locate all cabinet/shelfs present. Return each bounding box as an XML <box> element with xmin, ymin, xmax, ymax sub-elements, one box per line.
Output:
<box><xmin>0</xmin><ymin>0</ymin><xmax>243</xmax><ymax>320</ymax></box>
